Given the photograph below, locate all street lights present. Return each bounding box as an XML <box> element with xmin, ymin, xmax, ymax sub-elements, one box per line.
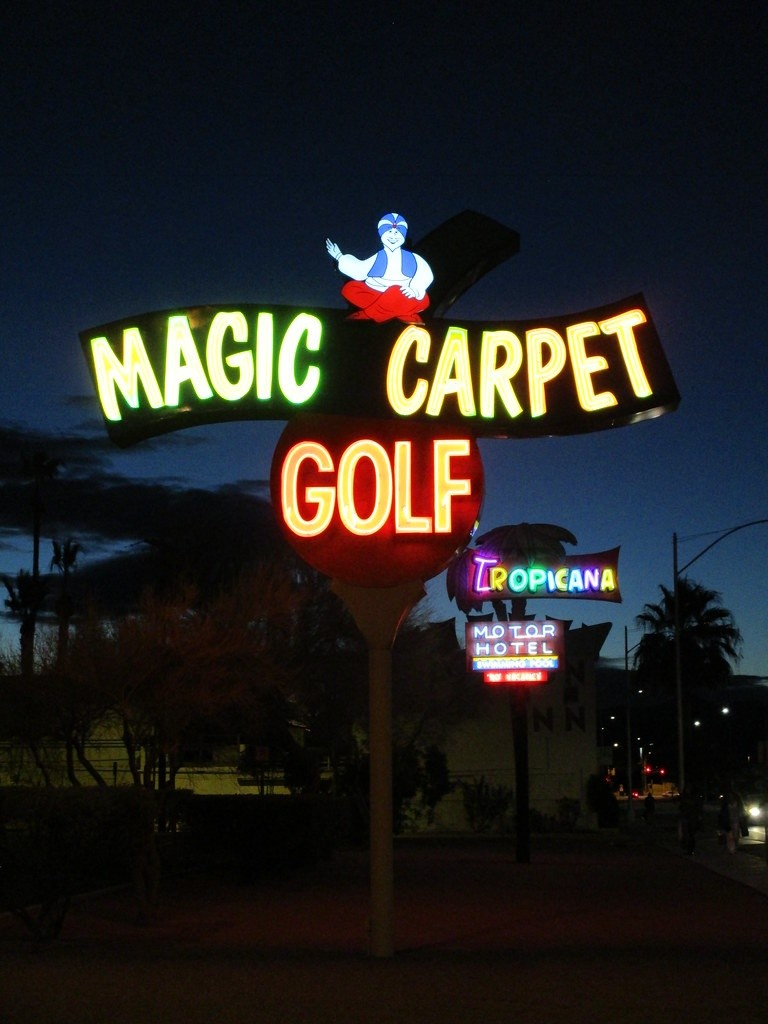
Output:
<box><xmin>597</xmin><ymin>682</ymin><xmax>734</xmax><ymax>819</ymax></box>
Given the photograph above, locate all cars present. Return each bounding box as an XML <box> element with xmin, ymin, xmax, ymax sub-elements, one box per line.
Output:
<box><xmin>727</xmin><ymin>781</ymin><xmax>767</xmax><ymax>844</ymax></box>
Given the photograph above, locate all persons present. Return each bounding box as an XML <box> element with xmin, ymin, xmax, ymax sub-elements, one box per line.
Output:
<box><xmin>644</xmin><ymin>793</ymin><xmax>655</xmax><ymax>816</ymax></box>
<box><xmin>719</xmin><ymin>792</ymin><xmax>741</xmax><ymax>855</ymax></box>
